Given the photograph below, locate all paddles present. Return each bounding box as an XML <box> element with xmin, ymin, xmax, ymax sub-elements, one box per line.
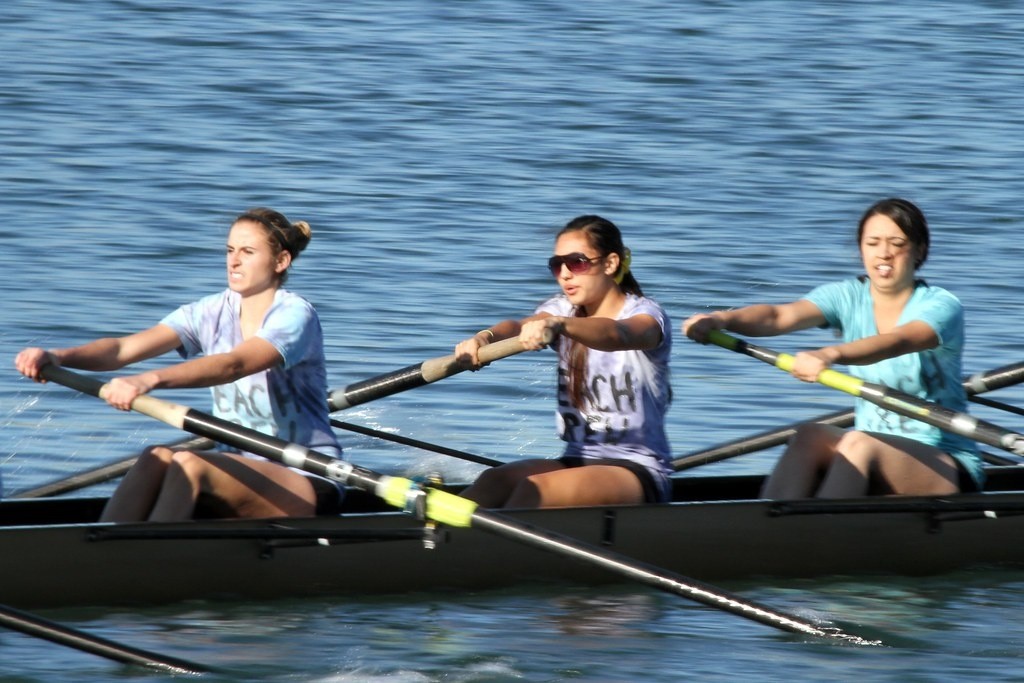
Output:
<box><xmin>1</xmin><ymin>325</ymin><xmax>1024</xmax><ymax>683</ymax></box>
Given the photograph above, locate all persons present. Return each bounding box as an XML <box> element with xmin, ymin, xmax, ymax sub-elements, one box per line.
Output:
<box><xmin>14</xmin><ymin>206</ymin><xmax>343</xmax><ymax>522</ymax></box>
<box><xmin>454</xmin><ymin>215</ymin><xmax>677</xmax><ymax>508</ymax></box>
<box><xmin>681</xmin><ymin>198</ymin><xmax>984</xmax><ymax>500</ymax></box>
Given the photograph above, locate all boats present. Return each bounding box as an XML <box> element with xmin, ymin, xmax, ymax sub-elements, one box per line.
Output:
<box><xmin>0</xmin><ymin>457</ymin><xmax>1024</xmax><ymax>620</ymax></box>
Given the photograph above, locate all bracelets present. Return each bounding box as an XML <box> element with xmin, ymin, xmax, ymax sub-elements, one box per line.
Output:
<box><xmin>475</xmin><ymin>328</ymin><xmax>495</xmax><ymax>337</ymax></box>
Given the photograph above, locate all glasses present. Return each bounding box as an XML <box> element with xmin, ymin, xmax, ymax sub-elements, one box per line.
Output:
<box><xmin>548</xmin><ymin>252</ymin><xmax>611</xmax><ymax>277</ymax></box>
